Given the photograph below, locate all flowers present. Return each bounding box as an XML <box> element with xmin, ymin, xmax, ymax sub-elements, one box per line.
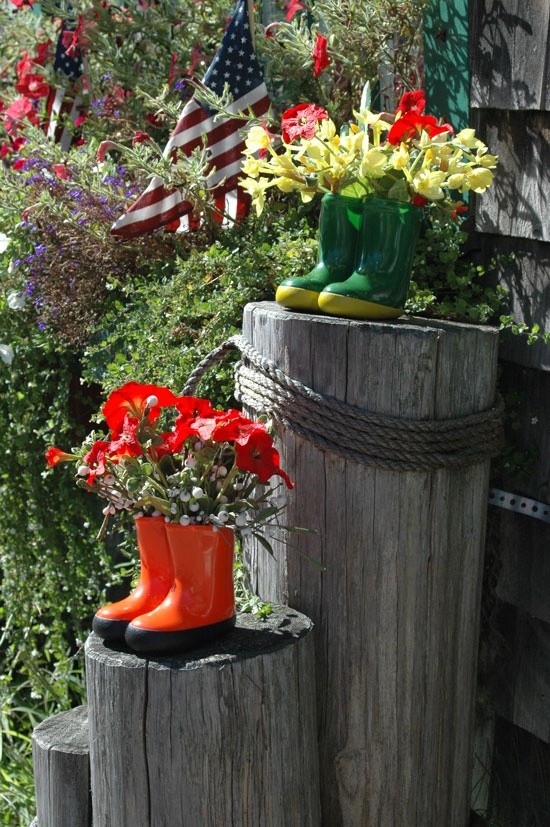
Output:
<box><xmin>183</xmin><ymin>34</ymin><xmax>500</xmax><ymax>219</ymax></box>
<box><xmin>45</xmin><ymin>381</ymin><xmax>329</xmax><ymax>574</ymax></box>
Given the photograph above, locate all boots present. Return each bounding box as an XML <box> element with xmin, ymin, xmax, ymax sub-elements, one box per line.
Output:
<box><xmin>125</xmin><ymin>524</ymin><xmax>237</xmax><ymax>651</ymax></box>
<box><xmin>317</xmin><ymin>198</ymin><xmax>424</xmax><ymax>317</ymax></box>
<box><xmin>275</xmin><ymin>193</ymin><xmax>363</xmax><ymax>309</ymax></box>
<box><xmin>92</xmin><ymin>516</ymin><xmax>175</xmax><ymax>638</ymax></box>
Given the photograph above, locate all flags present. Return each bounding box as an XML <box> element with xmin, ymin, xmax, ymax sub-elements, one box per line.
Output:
<box><xmin>108</xmin><ymin>2</ymin><xmax>277</xmax><ymax>239</ymax></box>
<box><xmin>35</xmin><ymin>20</ymin><xmax>96</xmax><ymax>153</ymax></box>
